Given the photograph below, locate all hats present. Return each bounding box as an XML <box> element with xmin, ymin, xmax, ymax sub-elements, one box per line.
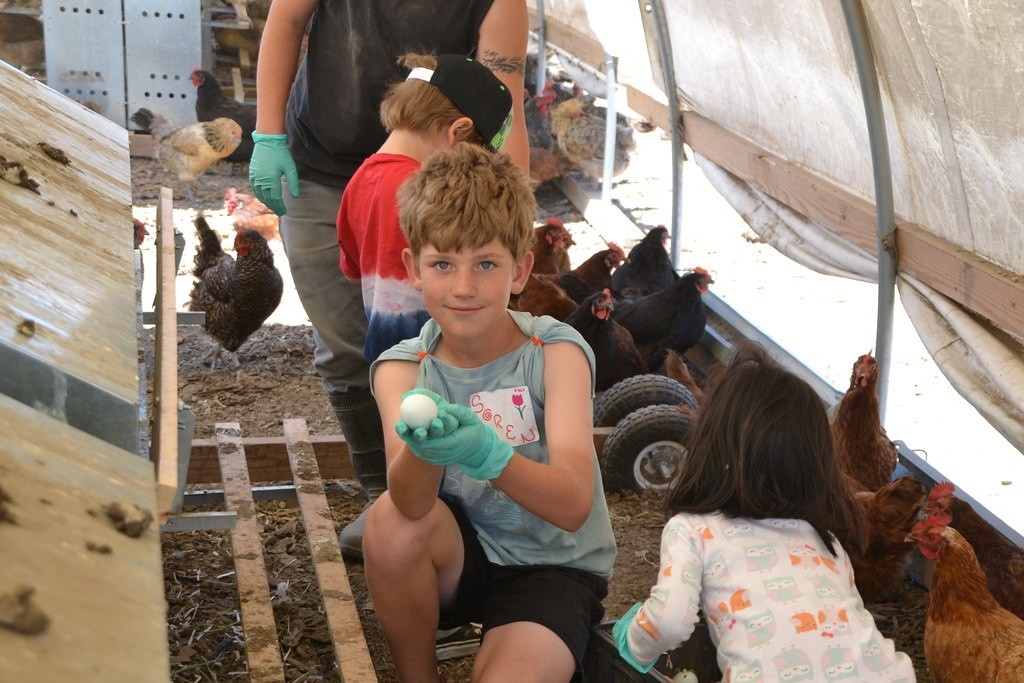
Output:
<box><xmin>405</xmin><ymin>53</ymin><xmax>515</xmax><ymax>154</ymax></box>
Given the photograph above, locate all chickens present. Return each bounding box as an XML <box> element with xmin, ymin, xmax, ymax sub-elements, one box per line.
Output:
<box><xmin>522</xmin><ymin>79</ymin><xmax>637</xmax><ymax>194</ymax></box>
<box><xmin>130</xmin><ymin>67</ymin><xmax>283</xmax><ymax>383</ymax></box>
<box><xmin>509</xmin><ymin>215</ymin><xmax>1024</xmax><ymax>683</ymax></box>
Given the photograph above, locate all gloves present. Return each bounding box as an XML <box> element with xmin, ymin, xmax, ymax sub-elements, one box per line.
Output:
<box><xmin>612</xmin><ymin>602</ymin><xmax>660</xmax><ymax>674</ymax></box>
<box><xmin>248</xmin><ymin>131</ymin><xmax>300</xmax><ymax>217</ymax></box>
<box><xmin>396</xmin><ymin>388</ymin><xmax>515</xmax><ymax>480</ymax></box>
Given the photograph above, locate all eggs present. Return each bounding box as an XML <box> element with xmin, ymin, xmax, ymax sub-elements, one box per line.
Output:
<box><xmin>399</xmin><ymin>394</ymin><xmax>437</xmax><ymax>429</ymax></box>
<box><xmin>672</xmin><ymin>671</ymin><xmax>698</xmax><ymax>683</ymax></box>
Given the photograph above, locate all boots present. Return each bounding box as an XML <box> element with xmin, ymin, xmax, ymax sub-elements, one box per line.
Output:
<box><xmin>328</xmin><ymin>389</ymin><xmax>388</xmax><ymax>557</ymax></box>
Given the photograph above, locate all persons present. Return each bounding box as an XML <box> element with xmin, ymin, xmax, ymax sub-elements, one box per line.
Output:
<box><xmin>247</xmin><ymin>0</ymin><xmax>534</xmax><ymax>551</ymax></box>
<box><xmin>611</xmin><ymin>341</ymin><xmax>917</xmax><ymax>683</ymax></box>
<box><xmin>335</xmin><ymin>50</ymin><xmax>516</xmax><ymax>663</ymax></box>
<box><xmin>362</xmin><ymin>146</ymin><xmax>618</xmax><ymax>683</ymax></box>
